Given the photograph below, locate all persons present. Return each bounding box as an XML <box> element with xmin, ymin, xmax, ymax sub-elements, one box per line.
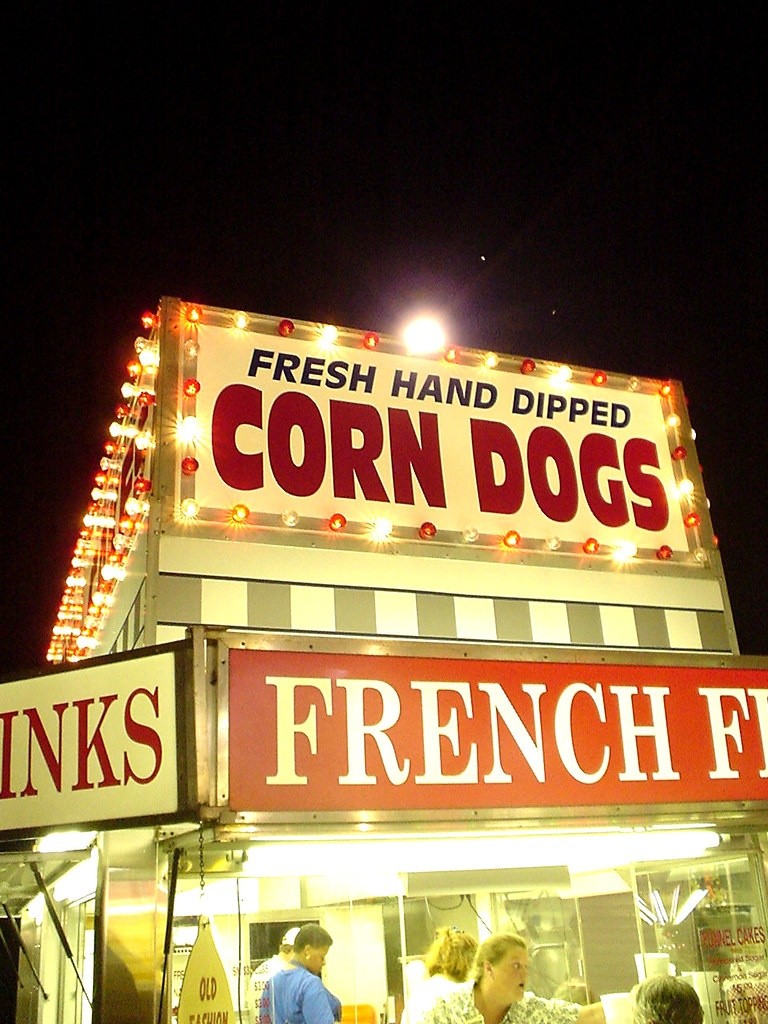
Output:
<box><xmin>420</xmin><ymin>932</ymin><xmax>605</xmax><ymax>1024</ymax></box>
<box><xmin>400</xmin><ymin>927</ymin><xmax>476</xmax><ymax>1024</ymax></box>
<box><xmin>251</xmin><ymin>927</ymin><xmax>300</xmax><ymax>1024</ymax></box>
<box><xmin>259</xmin><ymin>924</ymin><xmax>342</xmax><ymax>1023</ymax></box>
<box><xmin>633</xmin><ymin>975</ymin><xmax>705</xmax><ymax>1024</ymax></box>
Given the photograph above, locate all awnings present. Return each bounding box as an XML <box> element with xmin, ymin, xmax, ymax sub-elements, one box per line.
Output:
<box><xmin>0</xmin><ymin>848</ymin><xmax>93</xmax><ymax>1010</ymax></box>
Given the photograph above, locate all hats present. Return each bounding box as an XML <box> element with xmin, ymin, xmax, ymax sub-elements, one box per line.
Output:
<box><xmin>281</xmin><ymin>927</ymin><xmax>302</xmax><ymax>945</ymax></box>
